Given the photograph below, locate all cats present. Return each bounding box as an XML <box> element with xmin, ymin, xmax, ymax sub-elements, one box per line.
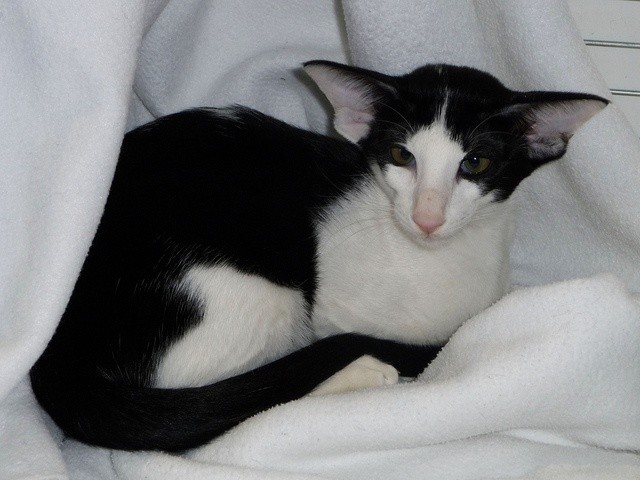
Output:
<box><xmin>30</xmin><ymin>60</ymin><xmax>609</xmax><ymax>453</ymax></box>
